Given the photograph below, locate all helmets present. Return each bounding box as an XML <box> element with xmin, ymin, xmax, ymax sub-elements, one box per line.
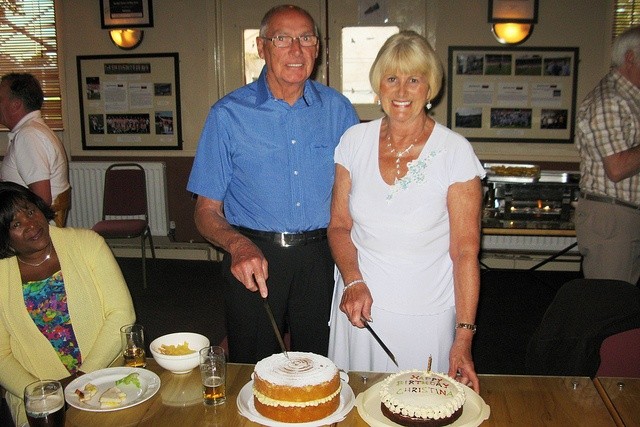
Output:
<box><xmin>64</xmin><ymin>367</ymin><xmax>162</xmax><ymax>412</ymax></box>
<box><xmin>354</xmin><ymin>380</ymin><xmax>491</xmax><ymax>427</ymax></box>
<box><xmin>236</xmin><ymin>371</ymin><xmax>355</xmax><ymax>427</ymax></box>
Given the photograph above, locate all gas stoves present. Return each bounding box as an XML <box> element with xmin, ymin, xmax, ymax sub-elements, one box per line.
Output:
<box><xmin>378</xmin><ymin>370</ymin><xmax>466</xmax><ymax>427</ymax></box>
<box><xmin>251</xmin><ymin>352</ymin><xmax>340</xmax><ymax>424</ymax></box>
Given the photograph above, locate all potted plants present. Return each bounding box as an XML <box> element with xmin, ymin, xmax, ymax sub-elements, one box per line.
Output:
<box><xmin>446</xmin><ymin>45</ymin><xmax>579</xmax><ymax>144</ymax></box>
<box><xmin>98</xmin><ymin>0</ymin><xmax>154</xmax><ymax>29</ymax></box>
<box><xmin>74</xmin><ymin>53</ymin><xmax>183</xmax><ymax>151</ymax></box>
<box><xmin>488</xmin><ymin>0</ymin><xmax>538</xmax><ymax>22</ymax></box>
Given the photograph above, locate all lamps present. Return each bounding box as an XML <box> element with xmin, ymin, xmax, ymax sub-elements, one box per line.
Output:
<box><xmin>522</xmin><ymin>279</ymin><xmax>640</xmax><ymax>377</ymax></box>
<box><xmin>91</xmin><ymin>163</ymin><xmax>157</xmax><ymax>289</ymax></box>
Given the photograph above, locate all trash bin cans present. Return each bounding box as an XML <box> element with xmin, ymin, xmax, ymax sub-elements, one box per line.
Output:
<box><xmin>454</xmin><ymin>322</ymin><xmax>478</xmax><ymax>334</ymax></box>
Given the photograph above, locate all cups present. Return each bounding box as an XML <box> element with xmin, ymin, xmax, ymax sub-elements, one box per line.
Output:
<box><xmin>260</xmin><ymin>35</ymin><xmax>318</xmax><ymax>49</ymax></box>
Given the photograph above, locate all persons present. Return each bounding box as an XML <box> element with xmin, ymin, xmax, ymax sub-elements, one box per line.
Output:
<box><xmin>1</xmin><ymin>182</ymin><xmax>136</xmax><ymax>426</ymax></box>
<box><xmin>0</xmin><ymin>73</ymin><xmax>70</xmax><ymax>205</ymax></box>
<box><xmin>185</xmin><ymin>4</ymin><xmax>358</xmax><ymax>363</ymax></box>
<box><xmin>326</xmin><ymin>30</ymin><xmax>487</xmax><ymax>399</ymax></box>
<box><xmin>573</xmin><ymin>26</ymin><xmax>639</xmax><ymax>281</ymax></box>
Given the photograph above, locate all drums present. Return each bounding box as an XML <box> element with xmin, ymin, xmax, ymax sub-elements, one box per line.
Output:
<box><xmin>361</xmin><ymin>317</ymin><xmax>398</xmax><ymax>368</ymax></box>
<box><xmin>251</xmin><ymin>273</ymin><xmax>290</xmax><ymax>358</ymax></box>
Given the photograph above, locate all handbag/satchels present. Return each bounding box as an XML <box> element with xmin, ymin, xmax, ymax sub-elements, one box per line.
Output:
<box><xmin>342</xmin><ymin>278</ymin><xmax>366</xmax><ymax>294</ymax></box>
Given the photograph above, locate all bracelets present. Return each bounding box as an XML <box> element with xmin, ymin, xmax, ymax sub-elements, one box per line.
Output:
<box><xmin>155</xmin><ymin>371</ymin><xmax>204</xmax><ymax>407</ymax></box>
<box><xmin>150</xmin><ymin>332</ymin><xmax>210</xmax><ymax>374</ymax></box>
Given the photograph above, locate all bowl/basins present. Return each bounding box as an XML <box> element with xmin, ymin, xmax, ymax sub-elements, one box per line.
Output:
<box><xmin>15</xmin><ymin>242</ymin><xmax>52</xmax><ymax>266</ymax></box>
<box><xmin>385</xmin><ymin>114</ymin><xmax>427</xmax><ymax>176</ymax></box>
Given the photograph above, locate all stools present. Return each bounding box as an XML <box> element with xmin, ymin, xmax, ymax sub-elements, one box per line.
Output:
<box><xmin>579</xmin><ymin>191</ymin><xmax>640</xmax><ymax>210</ymax></box>
<box><xmin>254</xmin><ymin>230</ymin><xmax>318</xmax><ymax>248</ymax></box>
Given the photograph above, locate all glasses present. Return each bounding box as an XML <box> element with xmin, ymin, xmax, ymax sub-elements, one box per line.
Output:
<box><xmin>109</xmin><ymin>29</ymin><xmax>144</xmax><ymax>50</ymax></box>
<box><xmin>490</xmin><ymin>23</ymin><xmax>535</xmax><ymax>44</ymax></box>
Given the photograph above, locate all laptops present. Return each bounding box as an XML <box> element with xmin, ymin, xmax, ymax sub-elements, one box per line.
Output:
<box><xmin>100</xmin><ymin>386</ymin><xmax>125</xmax><ymax>408</ymax></box>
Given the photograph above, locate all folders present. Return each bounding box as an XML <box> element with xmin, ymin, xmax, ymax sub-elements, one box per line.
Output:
<box><xmin>66</xmin><ymin>160</ymin><xmax>169</xmax><ymax>236</ymax></box>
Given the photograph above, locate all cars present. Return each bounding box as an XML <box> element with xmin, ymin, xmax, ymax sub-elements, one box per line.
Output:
<box><xmin>120</xmin><ymin>323</ymin><xmax>147</xmax><ymax>368</ymax></box>
<box><xmin>24</xmin><ymin>380</ymin><xmax>66</xmax><ymax>427</ymax></box>
<box><xmin>199</xmin><ymin>346</ymin><xmax>226</xmax><ymax>406</ymax></box>
